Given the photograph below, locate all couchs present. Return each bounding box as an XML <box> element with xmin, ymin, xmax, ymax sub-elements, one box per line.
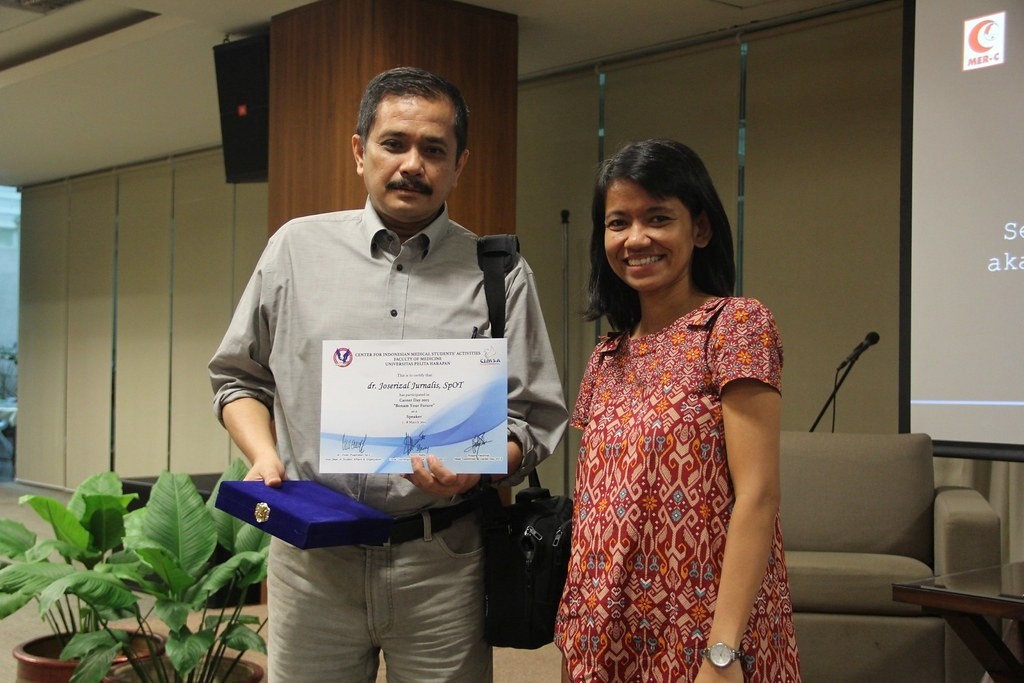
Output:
<box><xmin>779</xmin><ymin>432</ymin><xmax>1001</xmax><ymax>683</ymax></box>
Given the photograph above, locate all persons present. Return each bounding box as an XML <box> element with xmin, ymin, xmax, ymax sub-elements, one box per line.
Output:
<box><xmin>555</xmin><ymin>138</ymin><xmax>802</xmax><ymax>683</ymax></box>
<box><xmin>209</xmin><ymin>66</ymin><xmax>570</xmax><ymax>682</ymax></box>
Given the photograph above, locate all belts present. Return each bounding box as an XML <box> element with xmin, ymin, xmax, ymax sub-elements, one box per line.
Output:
<box><xmin>381</xmin><ymin>491</ymin><xmax>481</xmax><ymax>546</ymax></box>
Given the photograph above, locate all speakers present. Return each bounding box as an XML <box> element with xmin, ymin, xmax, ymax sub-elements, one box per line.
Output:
<box><xmin>213</xmin><ymin>33</ymin><xmax>270</xmax><ymax>184</ymax></box>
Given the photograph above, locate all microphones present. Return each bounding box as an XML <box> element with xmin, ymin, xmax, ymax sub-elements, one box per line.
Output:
<box><xmin>837</xmin><ymin>332</ymin><xmax>879</xmax><ymax>370</ymax></box>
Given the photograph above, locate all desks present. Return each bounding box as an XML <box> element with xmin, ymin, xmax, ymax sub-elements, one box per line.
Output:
<box><xmin>893</xmin><ymin>563</ymin><xmax>1024</xmax><ymax>683</ymax></box>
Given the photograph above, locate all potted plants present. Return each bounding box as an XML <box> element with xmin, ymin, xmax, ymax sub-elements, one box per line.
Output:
<box><xmin>0</xmin><ymin>458</ymin><xmax>270</xmax><ymax>683</ymax></box>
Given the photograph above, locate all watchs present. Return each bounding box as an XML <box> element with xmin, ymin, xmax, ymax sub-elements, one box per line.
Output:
<box><xmin>701</xmin><ymin>643</ymin><xmax>746</xmax><ymax>667</ymax></box>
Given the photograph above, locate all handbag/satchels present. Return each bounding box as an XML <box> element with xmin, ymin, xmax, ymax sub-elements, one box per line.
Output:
<box><xmin>482</xmin><ymin>490</ymin><xmax>576</xmax><ymax>649</ymax></box>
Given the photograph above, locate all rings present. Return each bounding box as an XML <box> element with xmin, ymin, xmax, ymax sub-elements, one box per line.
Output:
<box><xmin>449</xmin><ymin>493</ymin><xmax>456</xmax><ymax>503</ymax></box>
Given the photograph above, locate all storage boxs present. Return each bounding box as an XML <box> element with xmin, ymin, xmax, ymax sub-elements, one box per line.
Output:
<box><xmin>215</xmin><ymin>480</ymin><xmax>394</xmax><ymax>550</ymax></box>
